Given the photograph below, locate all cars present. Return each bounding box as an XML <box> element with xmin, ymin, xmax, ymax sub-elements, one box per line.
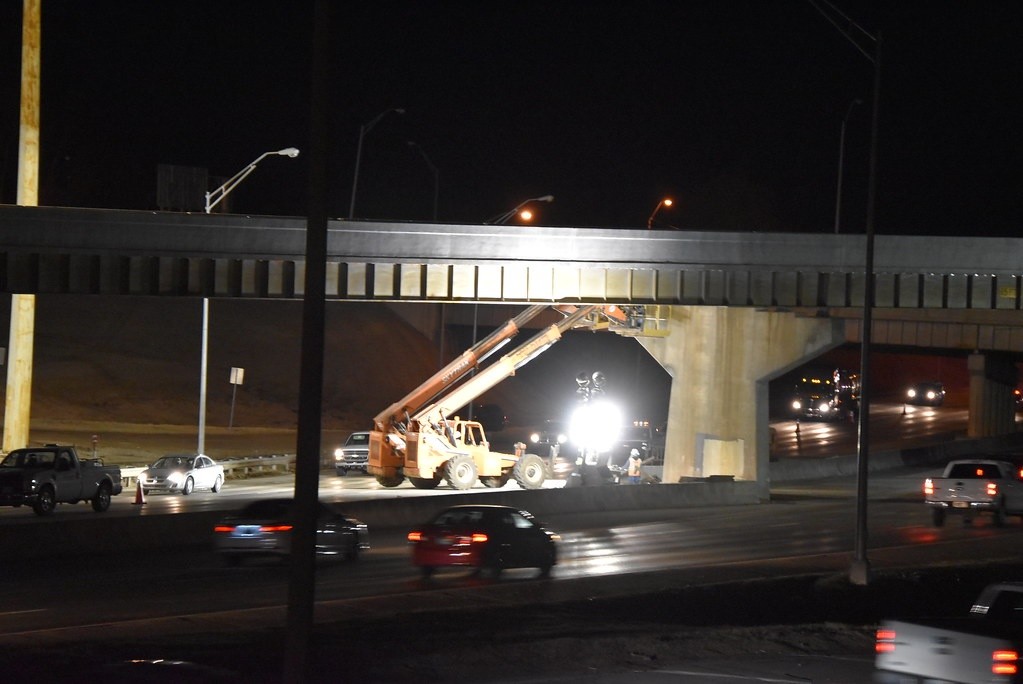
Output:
<box><xmin>906</xmin><ymin>383</ymin><xmax>944</xmax><ymax>406</ymax></box>
<box><xmin>213</xmin><ymin>496</ymin><xmax>369</xmax><ymax>567</ymax></box>
<box><xmin>138</xmin><ymin>453</ymin><xmax>225</xmax><ymax>495</ymax></box>
<box><xmin>407</xmin><ymin>505</ymin><xmax>559</xmax><ymax>579</ymax></box>
<box><xmin>448</xmin><ymin>404</ymin><xmax>507</xmax><ymax>433</ymax></box>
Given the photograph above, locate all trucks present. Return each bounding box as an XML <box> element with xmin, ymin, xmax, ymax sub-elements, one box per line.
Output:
<box><xmin>872</xmin><ymin>582</ymin><xmax>1023</xmax><ymax>684</ymax></box>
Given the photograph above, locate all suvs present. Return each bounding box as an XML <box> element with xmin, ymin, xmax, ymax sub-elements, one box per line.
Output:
<box><xmin>334</xmin><ymin>433</ymin><xmax>375</xmax><ymax>476</ymax></box>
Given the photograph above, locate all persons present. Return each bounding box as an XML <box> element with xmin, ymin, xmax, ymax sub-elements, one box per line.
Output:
<box><xmin>621</xmin><ymin>448</ymin><xmax>642</xmax><ymax>485</ymax></box>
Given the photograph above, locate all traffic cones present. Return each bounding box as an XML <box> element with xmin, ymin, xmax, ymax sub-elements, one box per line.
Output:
<box><xmin>902</xmin><ymin>404</ymin><xmax>908</xmax><ymax>415</ymax></box>
<box><xmin>847</xmin><ymin>410</ymin><xmax>855</xmax><ymax>424</ymax></box>
<box><xmin>131</xmin><ymin>478</ymin><xmax>148</xmax><ymax>504</ymax></box>
<box><xmin>795</xmin><ymin>418</ymin><xmax>802</xmax><ymax>433</ymax></box>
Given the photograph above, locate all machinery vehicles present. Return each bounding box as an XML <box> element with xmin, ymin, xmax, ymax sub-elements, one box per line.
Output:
<box><xmin>367</xmin><ymin>299</ymin><xmax>675</xmax><ymax>494</ymax></box>
<box><xmin>782</xmin><ymin>363</ymin><xmax>861</xmax><ymax>423</ymax></box>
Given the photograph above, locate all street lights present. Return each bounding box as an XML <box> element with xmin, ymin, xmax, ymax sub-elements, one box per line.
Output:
<box><xmin>404</xmin><ymin>139</ymin><xmax>439</xmax><ymax>222</ymax></box>
<box><xmin>349</xmin><ymin>107</ymin><xmax>407</xmax><ymax>218</ymax></box>
<box><xmin>468</xmin><ymin>195</ymin><xmax>554</xmax><ymax>441</ymax></box>
<box><xmin>194</xmin><ymin>148</ymin><xmax>301</xmax><ymax>454</ymax></box>
<box><xmin>646</xmin><ymin>199</ymin><xmax>674</xmax><ymax>230</ymax></box>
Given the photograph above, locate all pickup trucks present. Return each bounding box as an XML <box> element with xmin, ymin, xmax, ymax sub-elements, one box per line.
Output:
<box><xmin>0</xmin><ymin>445</ymin><xmax>123</xmax><ymax>516</ymax></box>
<box><xmin>924</xmin><ymin>459</ymin><xmax>1023</xmax><ymax>527</ymax></box>
